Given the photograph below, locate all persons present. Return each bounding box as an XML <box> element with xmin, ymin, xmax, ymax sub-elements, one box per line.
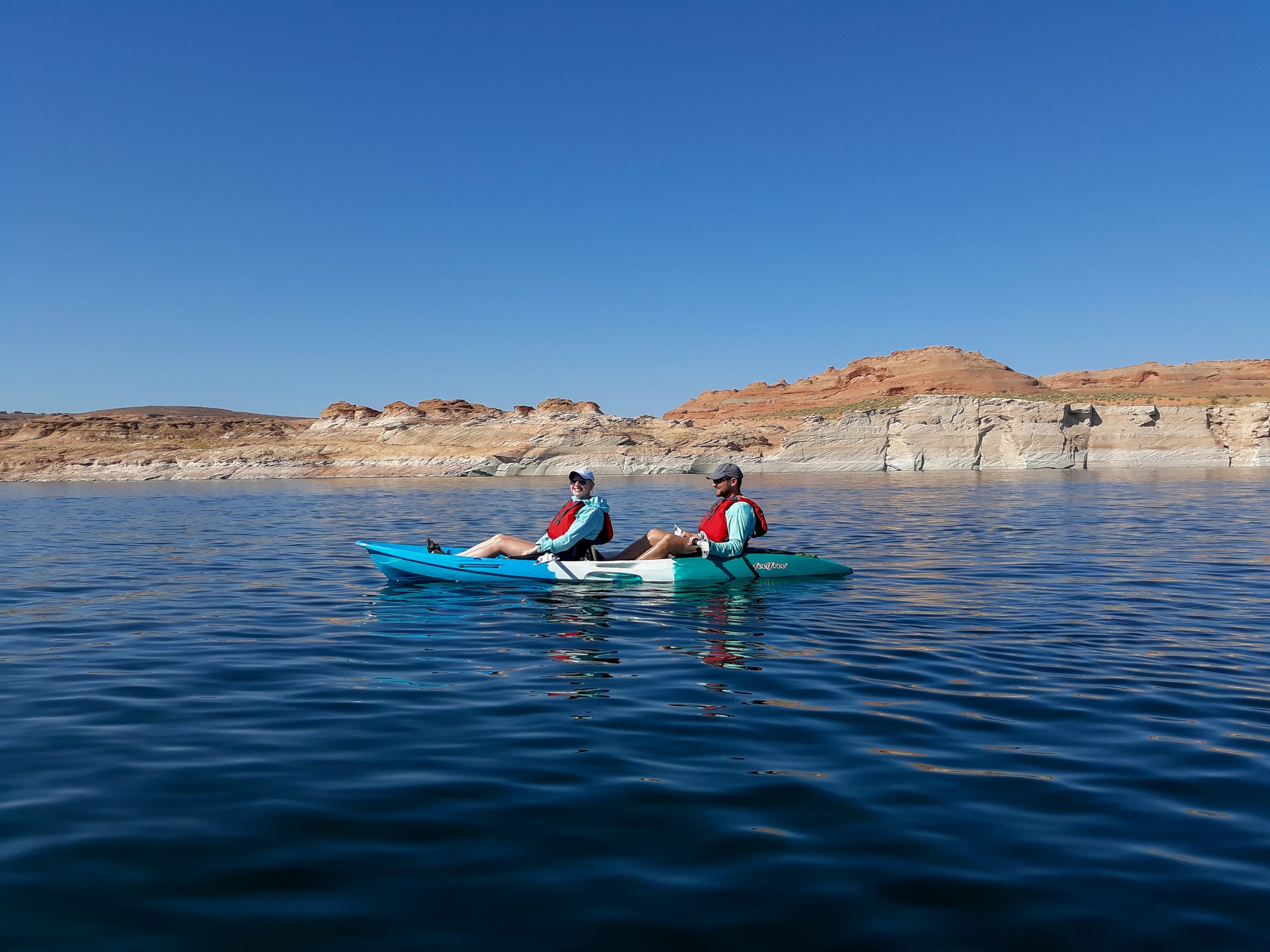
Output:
<box><xmin>426</xmin><ymin>467</ymin><xmax>610</xmax><ymax>561</ymax></box>
<box><xmin>595</xmin><ymin>463</ymin><xmax>756</xmax><ymax>561</ymax></box>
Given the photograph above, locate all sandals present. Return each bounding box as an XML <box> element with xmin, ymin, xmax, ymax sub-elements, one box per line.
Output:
<box><xmin>427</xmin><ymin>538</ymin><xmax>441</xmax><ymax>553</ymax></box>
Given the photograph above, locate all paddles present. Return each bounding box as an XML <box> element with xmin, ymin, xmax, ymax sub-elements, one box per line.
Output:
<box><xmin>673</xmin><ymin>524</ymin><xmax>711</xmax><ymax>559</ymax></box>
<box><xmin>533</xmin><ymin>550</ymin><xmax>561</xmax><ymax>566</ymax></box>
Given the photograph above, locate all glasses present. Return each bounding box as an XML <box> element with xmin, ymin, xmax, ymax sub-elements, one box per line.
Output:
<box><xmin>570</xmin><ymin>478</ymin><xmax>586</xmax><ymax>486</ymax></box>
<box><xmin>713</xmin><ymin>477</ymin><xmax>734</xmax><ymax>483</ymax></box>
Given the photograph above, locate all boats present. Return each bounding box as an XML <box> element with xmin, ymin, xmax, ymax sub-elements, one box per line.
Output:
<box><xmin>355</xmin><ymin>538</ymin><xmax>856</xmax><ymax>585</ymax></box>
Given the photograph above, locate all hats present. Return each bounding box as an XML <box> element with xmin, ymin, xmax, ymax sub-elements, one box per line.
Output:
<box><xmin>706</xmin><ymin>463</ymin><xmax>743</xmax><ymax>480</ymax></box>
<box><xmin>569</xmin><ymin>468</ymin><xmax>594</xmax><ymax>483</ymax></box>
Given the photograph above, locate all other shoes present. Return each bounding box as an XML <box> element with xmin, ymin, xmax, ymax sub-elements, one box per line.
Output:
<box><xmin>591</xmin><ymin>545</ymin><xmax>605</xmax><ymax>561</ymax></box>
